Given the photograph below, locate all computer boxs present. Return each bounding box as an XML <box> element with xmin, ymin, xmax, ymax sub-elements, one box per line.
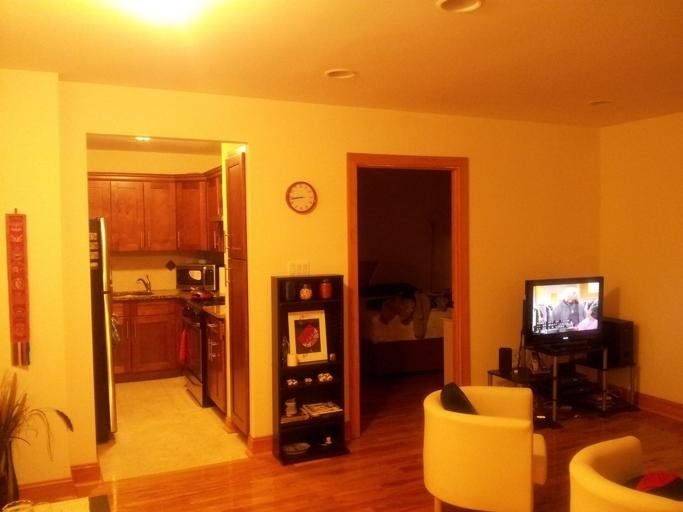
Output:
<box><xmin>586</xmin><ymin>317</ymin><xmax>635</xmax><ymax>368</ymax></box>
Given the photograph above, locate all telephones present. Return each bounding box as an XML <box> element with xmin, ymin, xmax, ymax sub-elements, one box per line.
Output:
<box><xmin>529</xmin><ymin>350</ymin><xmax>550</xmax><ymax>375</ymax></box>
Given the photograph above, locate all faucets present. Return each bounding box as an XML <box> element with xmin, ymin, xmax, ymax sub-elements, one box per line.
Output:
<box><xmin>134</xmin><ymin>272</ymin><xmax>152</xmax><ymax>293</ymax></box>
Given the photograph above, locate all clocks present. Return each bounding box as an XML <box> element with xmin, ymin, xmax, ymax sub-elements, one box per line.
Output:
<box><xmin>284</xmin><ymin>181</ymin><xmax>318</xmax><ymax>214</ymax></box>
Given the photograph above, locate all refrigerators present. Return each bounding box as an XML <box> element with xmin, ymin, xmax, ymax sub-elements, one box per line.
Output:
<box><xmin>88</xmin><ymin>216</ymin><xmax>117</xmax><ymax>443</ymax></box>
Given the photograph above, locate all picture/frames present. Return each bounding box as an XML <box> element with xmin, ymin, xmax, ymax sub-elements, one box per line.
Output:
<box><xmin>287</xmin><ymin>309</ymin><xmax>329</xmax><ymax>364</ymax></box>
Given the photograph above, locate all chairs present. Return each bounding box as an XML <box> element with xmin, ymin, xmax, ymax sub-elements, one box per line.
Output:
<box><xmin>420</xmin><ymin>383</ymin><xmax>549</xmax><ymax>512</ymax></box>
<box><xmin>569</xmin><ymin>436</ymin><xmax>683</xmax><ymax>512</ymax></box>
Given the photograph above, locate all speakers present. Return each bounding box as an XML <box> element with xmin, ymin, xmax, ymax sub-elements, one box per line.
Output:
<box><xmin>499</xmin><ymin>347</ymin><xmax>512</xmax><ymax>377</ymax></box>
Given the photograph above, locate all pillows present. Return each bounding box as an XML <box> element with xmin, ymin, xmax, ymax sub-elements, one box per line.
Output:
<box><xmin>440</xmin><ymin>384</ymin><xmax>480</xmax><ymax>415</ymax></box>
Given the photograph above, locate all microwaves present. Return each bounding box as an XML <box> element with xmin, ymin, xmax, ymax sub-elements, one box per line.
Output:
<box><xmin>175</xmin><ymin>263</ymin><xmax>218</xmax><ymax>291</ymax></box>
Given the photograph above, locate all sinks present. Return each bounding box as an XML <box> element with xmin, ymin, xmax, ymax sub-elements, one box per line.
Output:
<box><xmin>111</xmin><ymin>288</ymin><xmax>159</xmax><ymax>296</ymax></box>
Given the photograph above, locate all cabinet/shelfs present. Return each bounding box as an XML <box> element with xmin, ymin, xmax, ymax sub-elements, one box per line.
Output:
<box><xmin>183</xmin><ymin>298</ymin><xmax>226</xmax><ymax>414</ymax></box>
<box><xmin>87</xmin><ymin>165</ymin><xmax>222</xmax><ymax>255</ymax></box>
<box><xmin>486</xmin><ymin>316</ymin><xmax>636</xmax><ymax>430</ymax></box>
<box><xmin>113</xmin><ymin>290</ymin><xmax>184</xmax><ymax>385</ymax></box>
<box><xmin>270</xmin><ymin>271</ymin><xmax>350</xmax><ymax>469</ymax></box>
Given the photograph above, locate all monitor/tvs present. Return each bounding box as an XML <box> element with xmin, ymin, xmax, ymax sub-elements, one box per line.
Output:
<box><xmin>521</xmin><ymin>276</ymin><xmax>604</xmax><ymax>343</ymax></box>
